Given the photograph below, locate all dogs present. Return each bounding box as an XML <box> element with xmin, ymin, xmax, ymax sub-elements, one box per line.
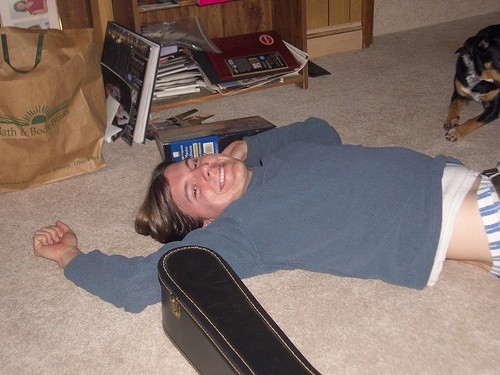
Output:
<box><xmin>443</xmin><ymin>23</ymin><xmax>500</xmax><ymax>143</ymax></box>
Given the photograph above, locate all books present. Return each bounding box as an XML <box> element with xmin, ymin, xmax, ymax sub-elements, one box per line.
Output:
<box><xmin>99</xmin><ymin>19</ymin><xmax>308</xmax><ymax>161</ymax></box>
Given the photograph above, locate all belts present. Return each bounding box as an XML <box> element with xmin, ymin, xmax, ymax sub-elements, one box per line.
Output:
<box><xmin>481</xmin><ymin>168</ymin><xmax>500</xmax><ymax>199</ymax></box>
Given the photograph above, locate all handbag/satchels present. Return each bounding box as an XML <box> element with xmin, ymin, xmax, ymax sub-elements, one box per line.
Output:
<box><xmin>1</xmin><ymin>27</ymin><xmax>108</xmax><ymax>194</ymax></box>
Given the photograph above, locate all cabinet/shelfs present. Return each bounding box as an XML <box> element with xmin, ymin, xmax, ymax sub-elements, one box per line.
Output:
<box><xmin>111</xmin><ymin>0</ymin><xmax>308</xmax><ymax>112</ymax></box>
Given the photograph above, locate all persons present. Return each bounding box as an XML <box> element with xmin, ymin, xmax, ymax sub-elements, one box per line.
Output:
<box><xmin>14</xmin><ymin>0</ymin><xmax>51</xmax><ymax>30</ymax></box>
<box><xmin>33</xmin><ymin>117</ymin><xmax>500</xmax><ymax>314</ymax></box>
